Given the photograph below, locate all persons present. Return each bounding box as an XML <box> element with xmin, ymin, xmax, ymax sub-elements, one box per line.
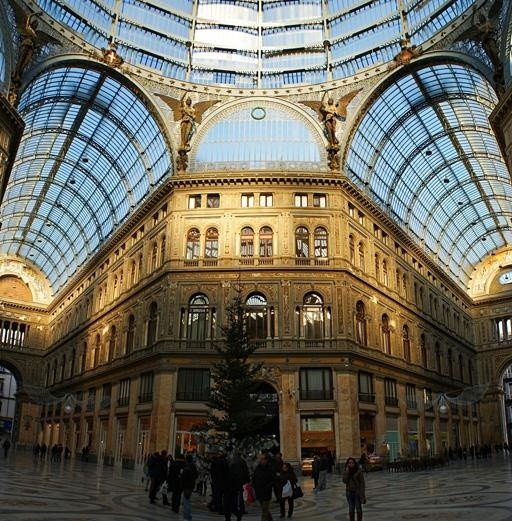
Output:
<box><xmin>444</xmin><ymin>441</ymin><xmax>512</xmax><ymax>466</ymax></box>
<box><xmin>469</xmin><ymin>1</ymin><xmax>504</xmax><ymax>76</ymax></box>
<box><xmin>33</xmin><ymin>443</ymin><xmax>40</xmax><ymax>459</ymax></box>
<box><xmin>10</xmin><ymin>11</ymin><xmax>42</xmax><ymax>87</ymax></box>
<box><xmin>143</xmin><ymin>447</ymin><xmax>367</xmax><ymax>521</ymax></box>
<box><xmin>2</xmin><ymin>437</ymin><xmax>11</xmax><ymax>458</ymax></box>
<box><xmin>319</xmin><ymin>91</ymin><xmax>342</xmax><ymax>148</ymax></box>
<box><xmin>64</xmin><ymin>446</ymin><xmax>70</xmax><ymax>459</ymax></box>
<box><xmin>55</xmin><ymin>444</ymin><xmax>63</xmax><ymax>462</ymax></box>
<box><xmin>178</xmin><ymin>91</ymin><xmax>197</xmax><ymax>149</ymax></box>
<box><xmin>50</xmin><ymin>444</ymin><xmax>57</xmax><ymax>462</ymax></box>
<box><xmin>41</xmin><ymin>443</ymin><xmax>47</xmax><ymax>458</ymax></box>
<box><xmin>82</xmin><ymin>445</ymin><xmax>86</xmax><ymax>457</ymax></box>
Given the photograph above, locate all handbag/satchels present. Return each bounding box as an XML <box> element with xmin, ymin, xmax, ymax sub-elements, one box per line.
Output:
<box><xmin>288</xmin><ymin>482</ymin><xmax>303</xmax><ymax>499</ymax></box>
